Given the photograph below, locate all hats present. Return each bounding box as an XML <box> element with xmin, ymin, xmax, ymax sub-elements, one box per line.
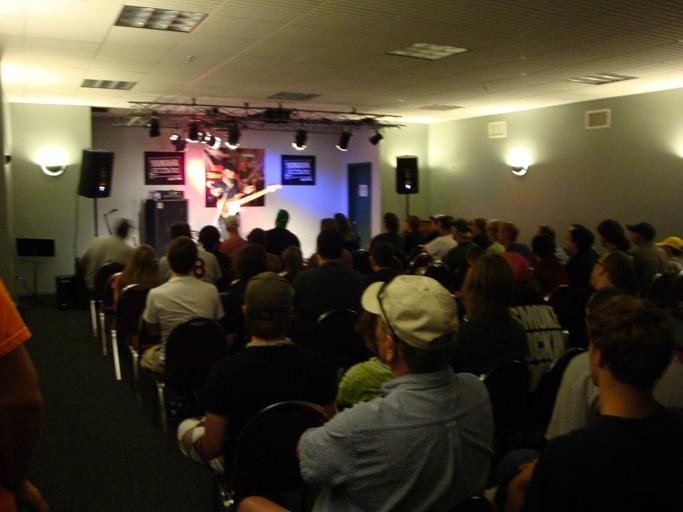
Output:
<box><xmin>429</xmin><ymin>213</ymin><xmax>444</xmax><ymax>221</ymax></box>
<box><xmin>246</xmin><ymin>271</ymin><xmax>296</xmax><ymax>319</ymax></box>
<box><xmin>626</xmin><ymin>221</ymin><xmax>656</xmax><ymax>238</ymax></box>
<box><xmin>655</xmin><ymin>236</ymin><xmax>683</xmax><ymax>252</ymax></box>
<box><xmin>361</xmin><ymin>274</ymin><xmax>459</xmax><ymax>351</ymax></box>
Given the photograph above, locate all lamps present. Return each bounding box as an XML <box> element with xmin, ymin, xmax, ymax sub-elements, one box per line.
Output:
<box><xmin>42</xmin><ymin>165</ymin><xmax>66</xmax><ymax>177</ymax></box>
<box><xmin>185</xmin><ymin>123</ymin><xmax>200</xmax><ymax>144</ymax></box>
<box><xmin>224</xmin><ymin>127</ymin><xmax>241</xmax><ymax>151</ymax></box>
<box><xmin>368</xmin><ymin>132</ymin><xmax>384</xmax><ymax>145</ymax></box>
<box><xmin>148</xmin><ymin>118</ymin><xmax>161</xmax><ymax>138</ymax></box>
<box><xmin>168</xmin><ymin>133</ymin><xmax>186</xmax><ymax>152</ymax></box>
<box><xmin>337</xmin><ymin>130</ymin><xmax>353</xmax><ymax>152</ymax></box>
<box><xmin>511</xmin><ymin>164</ymin><xmax>527</xmax><ymax>176</ymax></box>
<box><xmin>198</xmin><ymin>130</ymin><xmax>221</xmax><ymax>151</ymax></box>
<box><xmin>292</xmin><ymin>130</ymin><xmax>309</xmax><ymax>151</ymax></box>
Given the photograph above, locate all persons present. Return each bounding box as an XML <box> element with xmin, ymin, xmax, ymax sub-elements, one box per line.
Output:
<box><xmin>199</xmin><ymin>226</ymin><xmax>232</xmax><ymax>288</ymax></box>
<box><xmin>422</xmin><ymin>217</ymin><xmax>455</xmax><ymax>256</ymax></box>
<box><xmin>218</xmin><ymin>213</ymin><xmax>249</xmax><ymax>276</ymax></box>
<box><xmin>500</xmin><ymin>252</ymin><xmax>568</xmax><ymax>383</ymax></box>
<box><xmin>368</xmin><ymin>239</ymin><xmax>401</xmax><ymax>287</ymax></box>
<box><xmin>111</xmin><ymin>245</ymin><xmax>165</xmax><ymax>308</ymax></box>
<box><xmin>267</xmin><ymin>210</ymin><xmax>299</xmax><ymax>247</ymax></box>
<box><xmin>211</xmin><ymin>161</ymin><xmax>256</xmax><ymax>220</ymax></box>
<box><xmin>160</xmin><ymin>223</ymin><xmax>221</xmax><ymax>284</ymax></box>
<box><xmin>457</xmin><ymin>253</ymin><xmax>532</xmax><ymax>437</ymax></box>
<box><xmin>281</xmin><ymin>245</ymin><xmax>302</xmax><ymax>270</ymax></box>
<box><xmin>311</xmin><ymin>218</ymin><xmax>354</xmax><ymax>266</ymax></box>
<box><xmin>177</xmin><ymin>272</ymin><xmax>337</xmax><ymax>478</ymax></box>
<box><xmin>79</xmin><ymin>219</ymin><xmax>134</xmax><ymax>293</ymax></box>
<box><xmin>138</xmin><ymin>237</ymin><xmax>226</xmax><ymax>375</ymax></box>
<box><xmin>247</xmin><ymin>229</ymin><xmax>282</xmax><ymax>271</ymax></box>
<box><xmin>234</xmin><ymin>245</ymin><xmax>269</xmax><ymax>276</ymax></box>
<box><xmin>469</xmin><ymin>217</ymin><xmax>488</xmax><ymax>247</ymax></box>
<box><xmin>530</xmin><ymin>237</ymin><xmax>566</xmax><ymax>288</ymax></box>
<box><xmin>297</xmin><ymin>230</ymin><xmax>366</xmax><ymax>324</ymax></box>
<box><xmin>337</xmin><ymin>310</ymin><xmax>393</xmax><ymax>406</ymax></box>
<box><xmin>508</xmin><ymin>291</ymin><xmax>683</xmax><ymax>512</ymax></box>
<box><xmin>595</xmin><ymin>220</ymin><xmax>629</xmax><ymax>257</ymax></box>
<box><xmin>486</xmin><ymin>218</ymin><xmax>506</xmax><ymax>252</ymax></box>
<box><xmin>534</xmin><ymin>226</ymin><xmax>571</xmax><ymax>267</ymax></box>
<box><xmin>1</xmin><ymin>284</ymin><xmax>48</xmax><ymax>512</ymax></box>
<box><xmin>368</xmin><ymin>213</ymin><xmax>406</xmax><ymax>272</ymax></box>
<box><xmin>403</xmin><ymin>215</ymin><xmax>421</xmax><ymax>244</ymax></box>
<box><xmin>406</xmin><ymin>245</ymin><xmax>459</xmax><ymax>291</ymax></box>
<box><xmin>431</xmin><ymin>214</ymin><xmax>443</xmax><ymax>240</ymax></box>
<box><xmin>658</xmin><ymin>237</ymin><xmax>683</xmax><ymax>278</ymax></box>
<box><xmin>334</xmin><ymin>213</ymin><xmax>361</xmax><ymax>251</ymax></box>
<box><xmin>544</xmin><ymin>275</ymin><xmax>683</xmax><ymax>440</ymax></box>
<box><xmin>626</xmin><ymin>221</ymin><xmax>666</xmax><ymax>273</ymax></box>
<box><xmin>584</xmin><ymin>249</ymin><xmax>642</xmax><ymax>291</ymax></box>
<box><xmin>441</xmin><ymin>218</ymin><xmax>482</xmax><ymax>275</ymax></box>
<box><xmin>562</xmin><ymin>225</ymin><xmax>600</xmax><ymax>289</ymax></box>
<box><xmin>496</xmin><ymin>222</ymin><xmax>536</xmax><ymax>267</ymax></box>
<box><xmin>239</xmin><ymin>274</ymin><xmax>494</xmax><ymax>511</ymax></box>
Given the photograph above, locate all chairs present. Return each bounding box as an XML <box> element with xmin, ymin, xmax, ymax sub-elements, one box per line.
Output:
<box><xmin>127</xmin><ymin>313</ymin><xmax>146</xmax><ymax>408</ymax></box>
<box><xmin>548</xmin><ymin>347</ymin><xmax>585</xmax><ymax>373</ymax></box>
<box><xmin>317</xmin><ymin>308</ymin><xmax>361</xmax><ymax>324</ymax></box>
<box><xmin>211</xmin><ymin>399</ymin><xmax>331</xmax><ymax>512</ymax></box>
<box><xmin>477</xmin><ymin>360</ymin><xmax>533</xmax><ymax>394</ymax></box>
<box><xmin>151</xmin><ymin>316</ymin><xmax>226</xmax><ymax>433</ymax></box>
<box><xmin>110</xmin><ymin>282</ymin><xmax>143</xmax><ymax>381</ymax></box>
<box><xmin>98</xmin><ymin>269</ymin><xmax>124</xmax><ymax>358</ymax></box>
<box><xmin>546</xmin><ymin>283</ymin><xmax>570</xmax><ymax>304</ymax></box>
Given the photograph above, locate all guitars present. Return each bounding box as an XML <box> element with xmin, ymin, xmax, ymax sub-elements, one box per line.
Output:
<box><xmin>216</xmin><ymin>184</ymin><xmax>282</xmax><ymax>218</ymax></box>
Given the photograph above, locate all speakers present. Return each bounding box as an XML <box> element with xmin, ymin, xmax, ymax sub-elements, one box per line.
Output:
<box><xmin>145</xmin><ymin>198</ymin><xmax>188</xmax><ymax>257</ymax></box>
<box><xmin>397</xmin><ymin>155</ymin><xmax>419</xmax><ymax>194</ymax></box>
<box><xmin>78</xmin><ymin>148</ymin><xmax>114</xmax><ymax>198</ymax></box>
